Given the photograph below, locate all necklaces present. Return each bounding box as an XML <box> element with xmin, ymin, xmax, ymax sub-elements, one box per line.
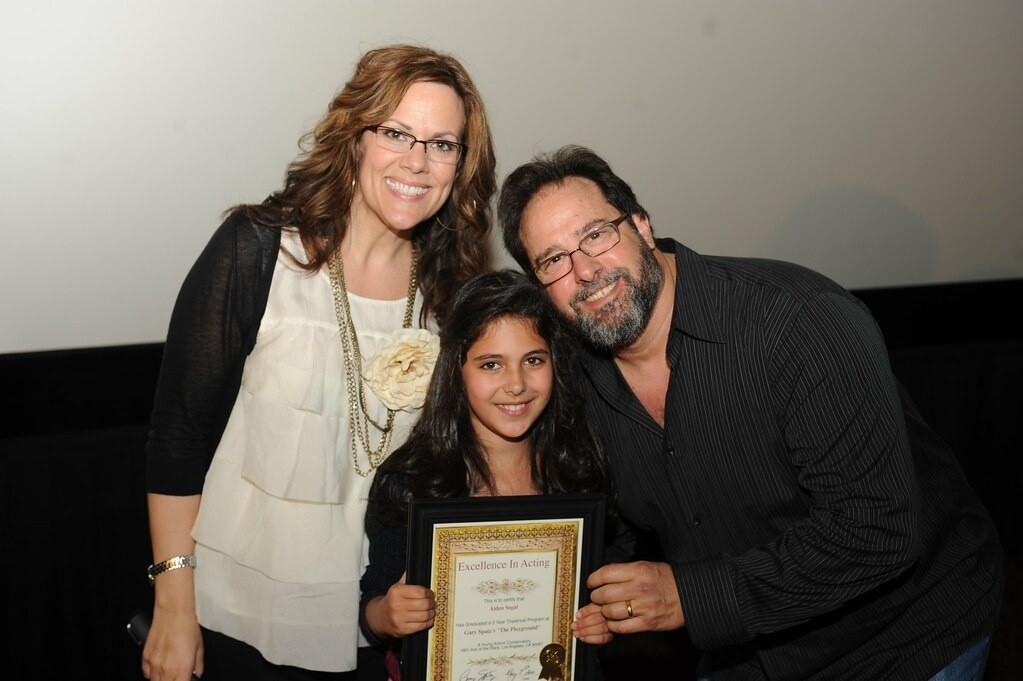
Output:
<box><xmin>328</xmin><ymin>245</ymin><xmax>420</xmax><ymax>476</ymax></box>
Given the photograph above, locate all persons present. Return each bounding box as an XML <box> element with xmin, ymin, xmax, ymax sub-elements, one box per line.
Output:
<box><xmin>358</xmin><ymin>270</ymin><xmax>639</xmax><ymax>681</ymax></box>
<box><xmin>497</xmin><ymin>147</ymin><xmax>1004</xmax><ymax>680</ymax></box>
<box><xmin>125</xmin><ymin>44</ymin><xmax>496</xmax><ymax>681</ymax></box>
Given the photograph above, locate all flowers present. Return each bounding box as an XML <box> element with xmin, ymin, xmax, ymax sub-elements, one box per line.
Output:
<box><xmin>361</xmin><ymin>327</ymin><xmax>441</xmax><ymax>415</ymax></box>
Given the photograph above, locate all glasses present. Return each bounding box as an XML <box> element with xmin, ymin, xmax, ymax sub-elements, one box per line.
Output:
<box><xmin>529</xmin><ymin>209</ymin><xmax>630</xmax><ymax>289</ymax></box>
<box><xmin>366</xmin><ymin>124</ymin><xmax>465</xmax><ymax>164</ymax></box>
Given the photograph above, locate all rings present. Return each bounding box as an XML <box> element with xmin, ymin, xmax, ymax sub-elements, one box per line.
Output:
<box><xmin>626</xmin><ymin>600</ymin><xmax>634</xmax><ymax>618</ymax></box>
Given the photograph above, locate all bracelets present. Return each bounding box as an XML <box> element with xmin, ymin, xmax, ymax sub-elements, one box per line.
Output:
<box><xmin>147</xmin><ymin>555</ymin><xmax>196</xmax><ymax>582</ymax></box>
